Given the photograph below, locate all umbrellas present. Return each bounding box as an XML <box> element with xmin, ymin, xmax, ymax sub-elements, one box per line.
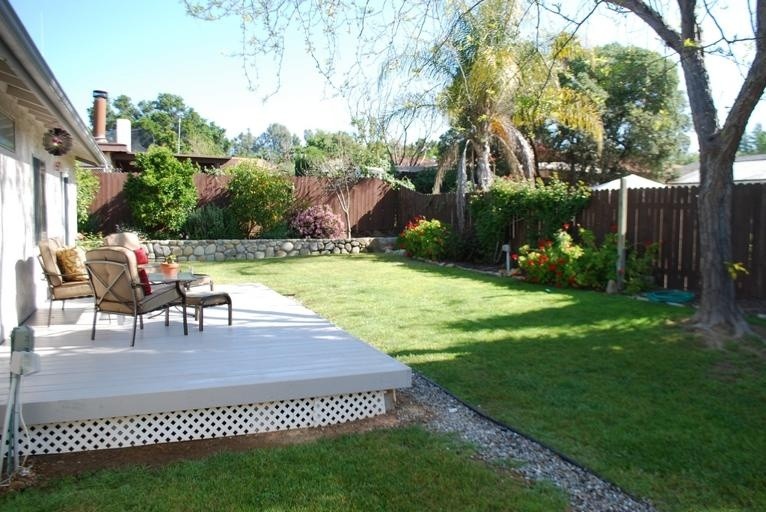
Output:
<box><xmin>593</xmin><ymin>171</ymin><xmax>667</xmax><ymax>188</ymax></box>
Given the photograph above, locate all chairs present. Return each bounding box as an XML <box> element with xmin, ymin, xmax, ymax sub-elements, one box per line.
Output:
<box><xmin>34</xmin><ymin>231</ymin><xmax>233</xmax><ymax>348</ymax></box>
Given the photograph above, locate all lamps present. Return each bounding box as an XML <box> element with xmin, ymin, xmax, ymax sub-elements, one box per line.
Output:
<box><xmin>43</xmin><ymin>128</ymin><xmax>73</xmax><ymax>156</ymax></box>
